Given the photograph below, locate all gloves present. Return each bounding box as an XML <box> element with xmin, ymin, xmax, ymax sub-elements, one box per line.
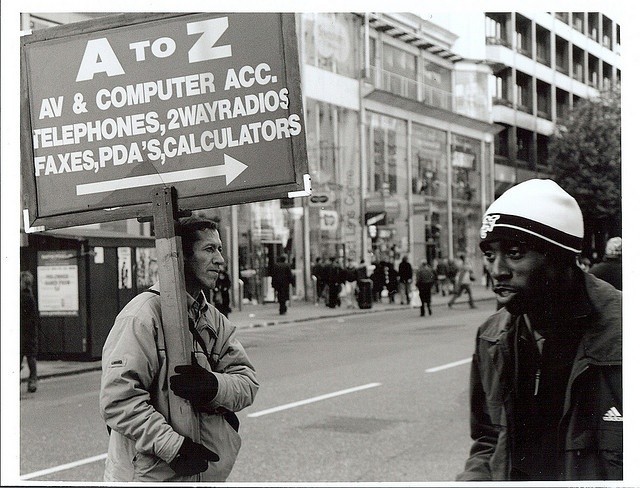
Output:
<box><xmin>170</xmin><ymin>353</ymin><xmax>219</xmax><ymax>404</ymax></box>
<box><xmin>167</xmin><ymin>436</ymin><xmax>220</xmax><ymax>477</ymax></box>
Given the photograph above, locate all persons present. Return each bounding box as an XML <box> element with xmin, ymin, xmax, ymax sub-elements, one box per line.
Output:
<box><xmin>436</xmin><ymin>260</ymin><xmax>447</xmax><ymax>296</ymax></box>
<box><xmin>214</xmin><ymin>265</ymin><xmax>230</xmax><ymax>319</ymax></box>
<box><xmin>588</xmin><ymin>237</ymin><xmax>622</xmax><ymax>290</ymax></box>
<box><xmin>483</xmin><ymin>265</ymin><xmax>494</xmax><ymax>289</ymax></box>
<box><xmin>448</xmin><ymin>258</ymin><xmax>458</xmax><ymax>283</ymax></box>
<box><xmin>272</xmin><ymin>254</ymin><xmax>296</xmax><ymax>315</ymax></box>
<box><xmin>20</xmin><ymin>270</ymin><xmax>41</xmax><ymax>393</ymax></box>
<box><xmin>289</xmin><ymin>257</ymin><xmax>296</xmax><ymax>287</ymax></box>
<box><xmin>416</xmin><ymin>260</ymin><xmax>434</xmax><ymax>315</ymax></box>
<box><xmin>455</xmin><ymin>178</ymin><xmax>623</xmax><ymax>479</ymax></box>
<box><xmin>310</xmin><ymin>254</ymin><xmax>413</xmax><ymax>309</ymax></box>
<box><xmin>447</xmin><ymin>254</ymin><xmax>477</xmax><ymax>308</ymax></box>
<box><xmin>99</xmin><ymin>213</ymin><xmax>260</xmax><ymax>481</ymax></box>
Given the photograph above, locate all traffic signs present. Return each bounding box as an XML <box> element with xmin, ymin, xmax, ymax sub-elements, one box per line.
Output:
<box><xmin>20</xmin><ymin>12</ymin><xmax>313</xmax><ymax>230</ymax></box>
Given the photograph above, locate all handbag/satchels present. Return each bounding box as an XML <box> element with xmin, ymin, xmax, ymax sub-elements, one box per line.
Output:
<box><xmin>207</xmin><ymin>407</ymin><xmax>240</xmax><ymax>433</ymax></box>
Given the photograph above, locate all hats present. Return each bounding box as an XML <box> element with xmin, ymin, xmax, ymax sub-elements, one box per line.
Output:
<box><xmin>605</xmin><ymin>237</ymin><xmax>622</xmax><ymax>256</ymax></box>
<box><xmin>479</xmin><ymin>178</ymin><xmax>584</xmax><ymax>260</ymax></box>
